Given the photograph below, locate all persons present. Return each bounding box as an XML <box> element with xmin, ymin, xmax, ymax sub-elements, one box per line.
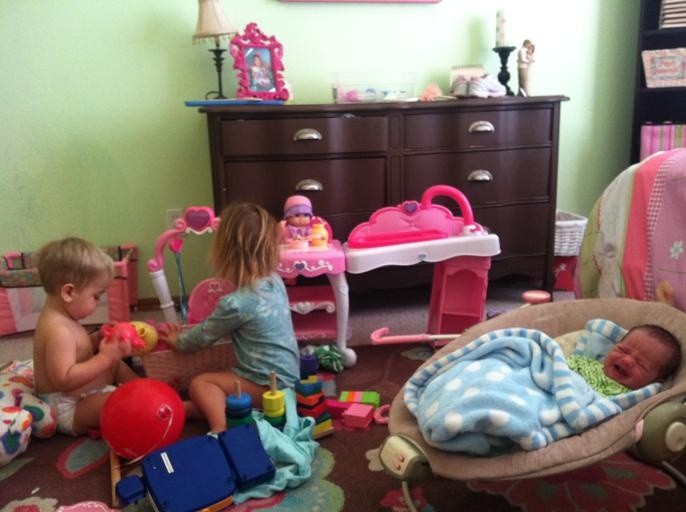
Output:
<box><xmin>32</xmin><ymin>236</ymin><xmax>141</xmax><ymax>438</ymax></box>
<box><xmin>157</xmin><ymin>201</ymin><xmax>301</xmax><ymax>433</ymax></box>
<box><xmin>251</xmin><ymin>53</ymin><xmax>272</xmax><ymax>88</ymax></box>
<box><xmin>274</xmin><ymin>193</ymin><xmax>328</xmax><ymax>247</ymax></box>
<box><xmin>567</xmin><ymin>323</ymin><xmax>681</xmax><ymax>396</ymax></box>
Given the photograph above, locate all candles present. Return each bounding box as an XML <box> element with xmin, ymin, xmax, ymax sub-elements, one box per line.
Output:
<box><xmin>494</xmin><ymin>10</ymin><xmax>509</xmax><ymax>49</ymax></box>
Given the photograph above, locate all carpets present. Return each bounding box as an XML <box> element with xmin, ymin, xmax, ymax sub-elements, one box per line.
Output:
<box><xmin>0</xmin><ymin>335</ymin><xmax>686</xmax><ymax>512</ymax></box>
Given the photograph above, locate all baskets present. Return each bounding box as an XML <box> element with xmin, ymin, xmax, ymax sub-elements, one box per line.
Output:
<box><xmin>556</xmin><ymin>210</ymin><xmax>587</xmax><ymax>257</ymax></box>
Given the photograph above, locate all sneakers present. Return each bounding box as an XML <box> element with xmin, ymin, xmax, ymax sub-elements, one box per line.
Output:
<box><xmin>452</xmin><ymin>74</ymin><xmax>506</xmax><ymax>98</ymax></box>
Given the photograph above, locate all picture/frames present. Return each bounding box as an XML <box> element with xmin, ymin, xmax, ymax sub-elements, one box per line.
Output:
<box><xmin>640</xmin><ymin>47</ymin><xmax>686</xmax><ymax>88</ymax></box>
<box><xmin>227</xmin><ymin>21</ymin><xmax>291</xmax><ymax>106</ymax></box>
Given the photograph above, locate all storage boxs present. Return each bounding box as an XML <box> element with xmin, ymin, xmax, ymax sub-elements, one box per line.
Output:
<box><xmin>526</xmin><ymin>256</ymin><xmax>578</xmax><ymax>294</ymax></box>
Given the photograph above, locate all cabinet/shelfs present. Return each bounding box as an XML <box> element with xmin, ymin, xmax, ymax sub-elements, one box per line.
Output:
<box><xmin>628</xmin><ymin>0</ymin><xmax>686</xmax><ymax>167</ymax></box>
<box><xmin>196</xmin><ymin>90</ymin><xmax>573</xmax><ymax>312</ymax></box>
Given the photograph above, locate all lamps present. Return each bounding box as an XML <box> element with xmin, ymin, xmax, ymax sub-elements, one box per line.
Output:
<box><xmin>190</xmin><ymin>0</ymin><xmax>240</xmax><ymax>104</ymax></box>
